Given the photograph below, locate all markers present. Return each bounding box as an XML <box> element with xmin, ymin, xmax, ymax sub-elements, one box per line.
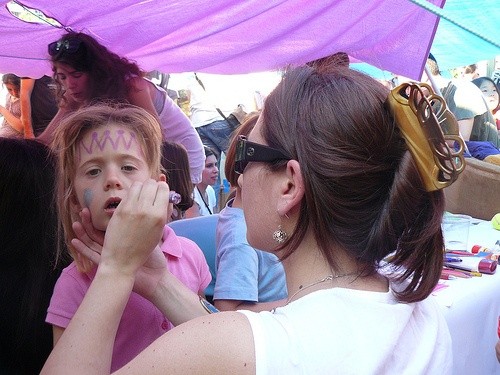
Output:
<box><xmin>169</xmin><ymin>190</ymin><xmax>181</xmax><ymax>204</ymax></box>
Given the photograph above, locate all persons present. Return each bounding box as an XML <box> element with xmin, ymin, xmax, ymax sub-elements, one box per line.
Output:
<box><xmin>0</xmin><ymin>31</ymin><xmax>500</xmax><ymax>375</ymax></box>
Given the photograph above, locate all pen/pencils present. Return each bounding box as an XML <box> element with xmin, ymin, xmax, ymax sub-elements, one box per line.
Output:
<box><xmin>438</xmin><ymin>249</ymin><xmax>482</xmax><ymax>281</ymax></box>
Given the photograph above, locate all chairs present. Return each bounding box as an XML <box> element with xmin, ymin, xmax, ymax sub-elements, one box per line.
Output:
<box><xmin>166</xmin><ymin>213</ymin><xmax>219</xmax><ymax>295</ymax></box>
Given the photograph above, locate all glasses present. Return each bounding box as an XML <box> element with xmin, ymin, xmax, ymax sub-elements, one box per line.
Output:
<box><xmin>233</xmin><ymin>134</ymin><xmax>287</xmax><ymax>174</ymax></box>
<box><xmin>48</xmin><ymin>38</ymin><xmax>82</xmax><ymax>56</ymax></box>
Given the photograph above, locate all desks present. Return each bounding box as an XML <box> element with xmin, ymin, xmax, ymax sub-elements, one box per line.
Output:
<box><xmin>378</xmin><ymin>213</ymin><xmax>500</xmax><ymax>375</ymax></box>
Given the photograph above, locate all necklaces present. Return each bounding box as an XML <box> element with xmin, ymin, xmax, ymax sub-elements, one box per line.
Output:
<box><xmin>284</xmin><ymin>272</ymin><xmax>360</xmax><ymax>302</ymax></box>
<box><xmin>198</xmin><ymin>185</ymin><xmax>215</xmax><ymax>215</ymax></box>
